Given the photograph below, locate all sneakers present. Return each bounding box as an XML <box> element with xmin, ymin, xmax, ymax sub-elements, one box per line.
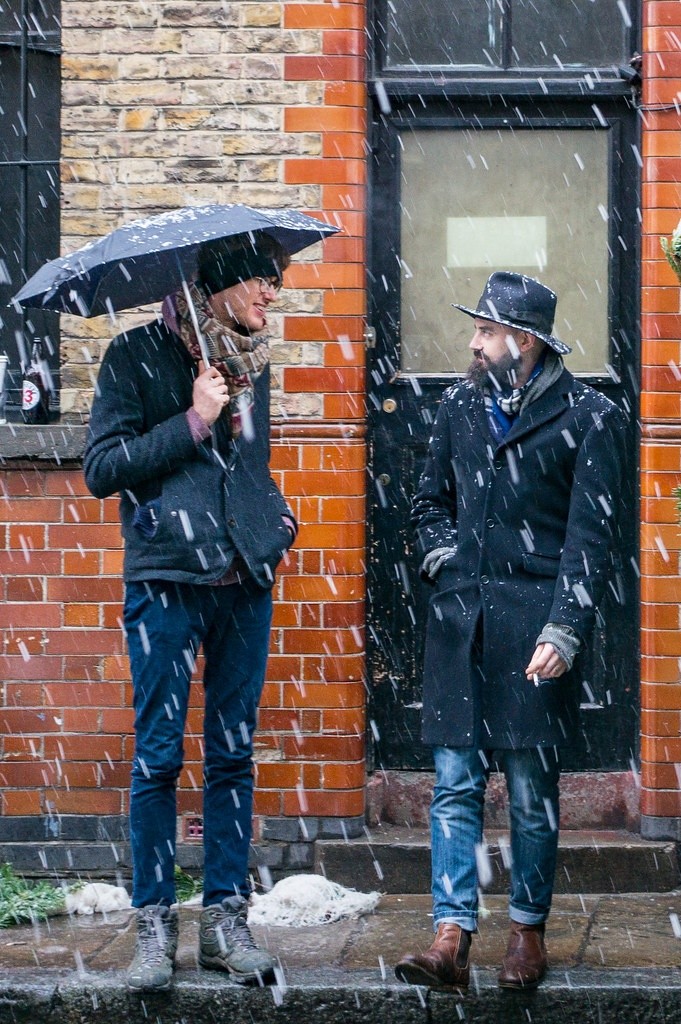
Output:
<box><xmin>125</xmin><ymin>898</ymin><xmax>178</xmax><ymax>992</ymax></box>
<box><xmin>198</xmin><ymin>895</ymin><xmax>279</xmax><ymax>984</ymax></box>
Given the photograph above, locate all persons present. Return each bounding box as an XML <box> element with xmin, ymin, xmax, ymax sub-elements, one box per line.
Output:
<box><xmin>394</xmin><ymin>271</ymin><xmax>629</xmax><ymax>993</ymax></box>
<box><xmin>83</xmin><ymin>229</ymin><xmax>300</xmax><ymax>991</ymax></box>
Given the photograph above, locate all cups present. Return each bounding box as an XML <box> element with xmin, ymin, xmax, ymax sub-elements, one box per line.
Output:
<box><xmin>0</xmin><ymin>356</ymin><xmax>8</xmax><ymax>393</ymax></box>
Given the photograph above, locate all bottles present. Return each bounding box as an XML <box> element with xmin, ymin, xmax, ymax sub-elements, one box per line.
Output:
<box><xmin>22</xmin><ymin>337</ymin><xmax>49</xmax><ymax>425</ymax></box>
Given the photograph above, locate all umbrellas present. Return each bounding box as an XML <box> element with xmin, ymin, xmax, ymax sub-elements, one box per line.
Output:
<box><xmin>10</xmin><ymin>203</ymin><xmax>343</xmax><ymax>455</ymax></box>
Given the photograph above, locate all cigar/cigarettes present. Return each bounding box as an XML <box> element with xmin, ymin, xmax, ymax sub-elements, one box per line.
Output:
<box><xmin>533</xmin><ymin>673</ymin><xmax>540</xmax><ymax>687</ymax></box>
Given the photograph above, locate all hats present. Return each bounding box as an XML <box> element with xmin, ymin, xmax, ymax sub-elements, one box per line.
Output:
<box><xmin>452</xmin><ymin>271</ymin><xmax>572</xmax><ymax>354</ymax></box>
<box><xmin>199</xmin><ymin>230</ymin><xmax>282</xmax><ymax>296</ymax></box>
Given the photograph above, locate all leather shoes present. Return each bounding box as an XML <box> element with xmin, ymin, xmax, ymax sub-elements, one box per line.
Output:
<box><xmin>394</xmin><ymin>922</ymin><xmax>472</xmax><ymax>996</ymax></box>
<box><xmin>498</xmin><ymin>917</ymin><xmax>548</xmax><ymax>990</ymax></box>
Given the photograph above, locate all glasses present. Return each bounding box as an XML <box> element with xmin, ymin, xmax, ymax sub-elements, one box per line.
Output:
<box><xmin>255</xmin><ymin>276</ymin><xmax>283</xmax><ymax>293</ymax></box>
